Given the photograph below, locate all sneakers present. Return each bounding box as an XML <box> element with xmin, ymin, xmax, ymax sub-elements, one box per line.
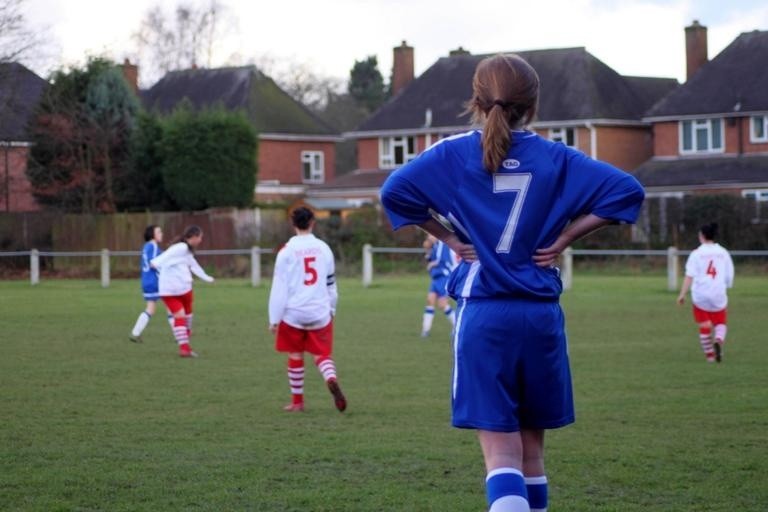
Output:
<box><xmin>180</xmin><ymin>348</ymin><xmax>197</xmax><ymax>357</ymax></box>
<box><xmin>712</xmin><ymin>341</ymin><xmax>722</xmax><ymax>361</ymax></box>
<box><xmin>283</xmin><ymin>403</ymin><xmax>302</xmax><ymax>410</ymax></box>
<box><xmin>327</xmin><ymin>379</ymin><xmax>345</xmax><ymax>410</ymax></box>
<box><xmin>129</xmin><ymin>333</ymin><xmax>143</xmax><ymax>344</ymax></box>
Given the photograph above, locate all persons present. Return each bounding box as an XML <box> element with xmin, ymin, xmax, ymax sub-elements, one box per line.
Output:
<box><xmin>128</xmin><ymin>225</ymin><xmax>178</xmax><ymax>344</ymax></box>
<box><xmin>676</xmin><ymin>222</ymin><xmax>736</xmax><ymax>362</ymax></box>
<box><xmin>268</xmin><ymin>207</ymin><xmax>347</xmax><ymax>412</ymax></box>
<box><xmin>421</xmin><ymin>231</ymin><xmax>456</xmax><ymax>338</ymax></box>
<box><xmin>377</xmin><ymin>54</ymin><xmax>646</xmax><ymax>511</ymax></box>
<box><xmin>152</xmin><ymin>224</ymin><xmax>216</xmax><ymax>357</ymax></box>
